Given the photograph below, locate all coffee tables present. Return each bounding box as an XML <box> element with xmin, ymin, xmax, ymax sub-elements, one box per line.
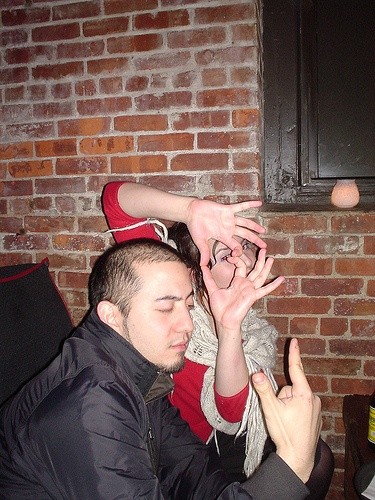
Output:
<box><xmin>343</xmin><ymin>394</ymin><xmax>375</xmax><ymax>500</ymax></box>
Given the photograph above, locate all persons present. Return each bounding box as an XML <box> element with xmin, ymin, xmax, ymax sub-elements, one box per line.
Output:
<box><xmin>1</xmin><ymin>237</ymin><xmax>323</xmax><ymax>500</ymax></box>
<box><xmin>101</xmin><ymin>181</ymin><xmax>335</xmax><ymax>500</ymax></box>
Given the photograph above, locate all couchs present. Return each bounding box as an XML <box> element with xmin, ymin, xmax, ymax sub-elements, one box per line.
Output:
<box><xmin>0</xmin><ymin>257</ymin><xmax>76</xmax><ymax>408</ymax></box>
